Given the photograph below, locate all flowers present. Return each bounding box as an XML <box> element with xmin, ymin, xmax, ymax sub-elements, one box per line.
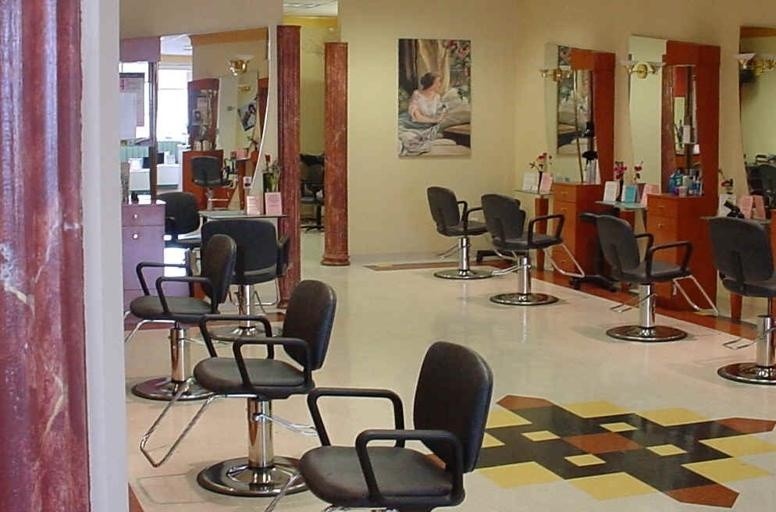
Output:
<box><xmin>613</xmin><ymin>160</ymin><xmax>627</xmax><ymax>182</ymax></box>
<box><xmin>633</xmin><ymin>161</ymin><xmax>645</xmax><ymax>183</ymax></box>
<box><xmin>747</xmin><ymin>54</ymin><xmax>774</xmax><ymax>76</ymax></box>
<box><xmin>529</xmin><ymin>152</ymin><xmax>553</xmax><ymax>173</ymax></box>
<box><xmin>716</xmin><ymin>168</ymin><xmax>736</xmax><ymax>194</ymax></box>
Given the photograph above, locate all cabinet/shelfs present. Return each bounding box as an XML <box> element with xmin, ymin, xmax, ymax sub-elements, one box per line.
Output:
<box><xmin>647</xmin><ymin>41</ymin><xmax>719</xmax><ymax>310</ymax></box>
<box><xmin>551</xmin><ymin>47</ymin><xmax>616</xmax><ymax>278</ymax></box>
<box><xmin>183</xmin><ymin>78</ymin><xmax>224</xmax><ymax>209</ymax></box>
<box><xmin>124</xmin><ymin>204</ymin><xmax>169</xmax><ymax>291</ymax></box>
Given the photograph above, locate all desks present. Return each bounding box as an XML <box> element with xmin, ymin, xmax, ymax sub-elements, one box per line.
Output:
<box><xmin>700</xmin><ymin>216</ymin><xmax>767</xmax><ymax>322</ymax></box>
<box><xmin>514</xmin><ymin>189</ymin><xmax>552</xmax><ymax>271</ymax></box>
<box><xmin>595</xmin><ymin>200</ymin><xmax>647</xmax><ymax>292</ymax></box>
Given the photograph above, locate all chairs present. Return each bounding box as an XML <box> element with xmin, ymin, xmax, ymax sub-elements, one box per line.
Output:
<box><xmin>200</xmin><ymin>216</ymin><xmax>296</xmax><ymax>347</ymax></box>
<box><xmin>261</xmin><ymin>340</ymin><xmax>493</xmax><ymax>512</ymax></box>
<box><xmin>152</xmin><ymin>192</ymin><xmax>202</xmax><ymax>252</ymax></box>
<box><xmin>596</xmin><ymin>215</ymin><xmax>717</xmax><ymax>342</ymax></box>
<box><xmin>123</xmin><ymin>233</ymin><xmax>240</xmax><ymax>402</ymax></box>
<box><xmin>138</xmin><ymin>280</ymin><xmax>337</xmax><ymax>501</ymax></box>
<box><xmin>482</xmin><ymin>194</ymin><xmax>586</xmax><ymax>306</ymax></box>
<box><xmin>707</xmin><ymin>216</ymin><xmax>776</xmax><ymax>385</ymax></box>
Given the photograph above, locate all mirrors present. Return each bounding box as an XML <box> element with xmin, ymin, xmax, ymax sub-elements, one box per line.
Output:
<box><xmin>545</xmin><ymin>44</ymin><xmax>597</xmax><ymax>185</ymax></box>
<box><xmin>118</xmin><ymin>34</ymin><xmax>269</xmax><ymax>206</ymax></box>
<box><xmin>627</xmin><ymin>34</ymin><xmax>662</xmax><ymax>193</ymax></box>
<box><xmin>739</xmin><ymin>35</ymin><xmax>775</xmax><ymax>214</ymax></box>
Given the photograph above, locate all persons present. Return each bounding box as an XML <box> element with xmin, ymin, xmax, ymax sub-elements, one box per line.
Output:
<box><xmin>408</xmin><ymin>73</ymin><xmax>449</xmax><ymax>124</ymax></box>
<box><xmin>244</xmin><ymin>104</ymin><xmax>256</xmax><ymax>127</ymax></box>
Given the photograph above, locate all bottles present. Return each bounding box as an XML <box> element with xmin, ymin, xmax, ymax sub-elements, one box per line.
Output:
<box><xmin>261</xmin><ymin>153</ymin><xmax>277</xmax><ymax>215</ymax></box>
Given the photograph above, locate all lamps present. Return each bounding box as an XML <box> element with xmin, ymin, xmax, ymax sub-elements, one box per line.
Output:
<box><xmin>733</xmin><ymin>52</ymin><xmax>755</xmax><ymax>84</ymax></box>
<box><xmin>620</xmin><ymin>52</ymin><xmax>647</xmax><ymax>80</ymax></box>
<box><xmin>539</xmin><ymin>66</ymin><xmax>565</xmax><ymax>83</ymax></box>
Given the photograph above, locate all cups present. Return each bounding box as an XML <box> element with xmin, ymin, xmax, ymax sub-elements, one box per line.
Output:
<box><xmin>193</xmin><ymin>138</ymin><xmax>213</xmax><ymax>152</ymax></box>
<box><xmin>623</xmin><ymin>186</ymin><xmax>636</xmax><ymax>203</ymax></box>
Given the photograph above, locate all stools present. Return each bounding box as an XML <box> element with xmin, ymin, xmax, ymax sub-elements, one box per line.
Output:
<box><xmin>476</xmin><ymin>201</ymin><xmax>525</xmax><ymax>263</ymax></box>
<box><xmin>569</xmin><ymin>212</ymin><xmax>621</xmax><ymax>293</ymax></box>
